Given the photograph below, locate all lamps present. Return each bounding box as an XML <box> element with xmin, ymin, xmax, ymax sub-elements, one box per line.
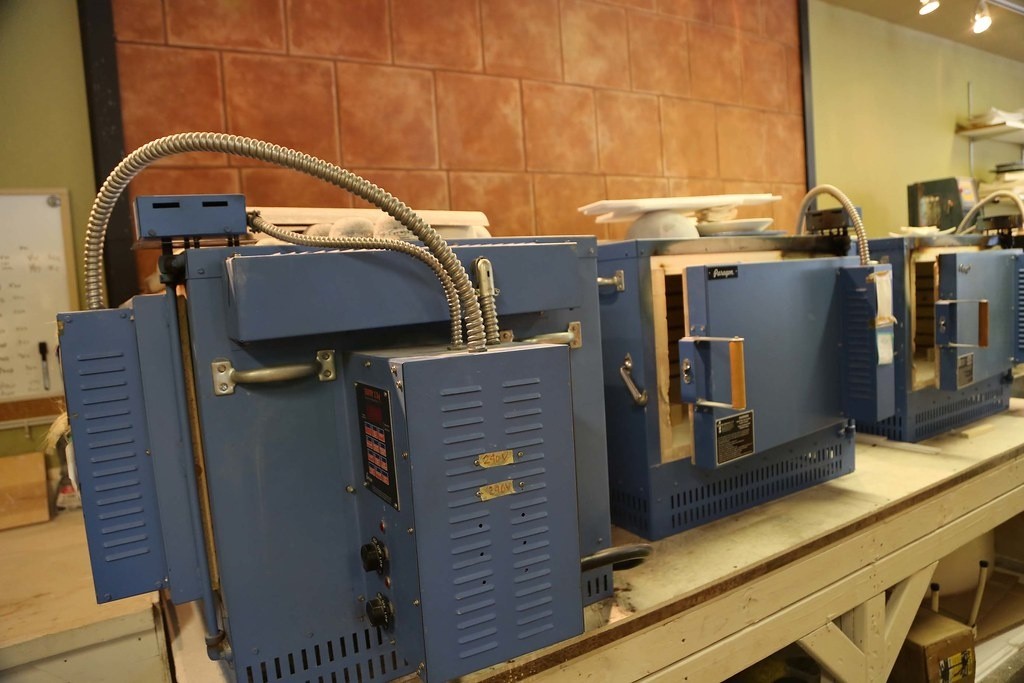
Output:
<box><xmin>917</xmin><ymin>0</ymin><xmax>939</xmax><ymax>15</ymax></box>
<box><xmin>972</xmin><ymin>0</ymin><xmax>992</xmax><ymax>35</ymax></box>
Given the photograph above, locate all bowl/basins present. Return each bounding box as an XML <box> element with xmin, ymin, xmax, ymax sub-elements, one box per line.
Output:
<box><xmin>627</xmin><ymin>210</ymin><xmax>701</xmax><ymax>238</ymax></box>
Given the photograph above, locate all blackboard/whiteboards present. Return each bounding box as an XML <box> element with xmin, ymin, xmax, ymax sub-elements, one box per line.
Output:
<box><xmin>0</xmin><ymin>187</ymin><xmax>80</xmax><ymax>405</ymax></box>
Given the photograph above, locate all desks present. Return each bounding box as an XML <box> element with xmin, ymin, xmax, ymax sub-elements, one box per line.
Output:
<box><xmin>0</xmin><ymin>398</ymin><xmax>1024</xmax><ymax>683</ymax></box>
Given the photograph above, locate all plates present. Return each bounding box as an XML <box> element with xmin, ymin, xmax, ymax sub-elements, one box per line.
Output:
<box><xmin>698</xmin><ymin>218</ymin><xmax>774</xmax><ymax>233</ymax></box>
